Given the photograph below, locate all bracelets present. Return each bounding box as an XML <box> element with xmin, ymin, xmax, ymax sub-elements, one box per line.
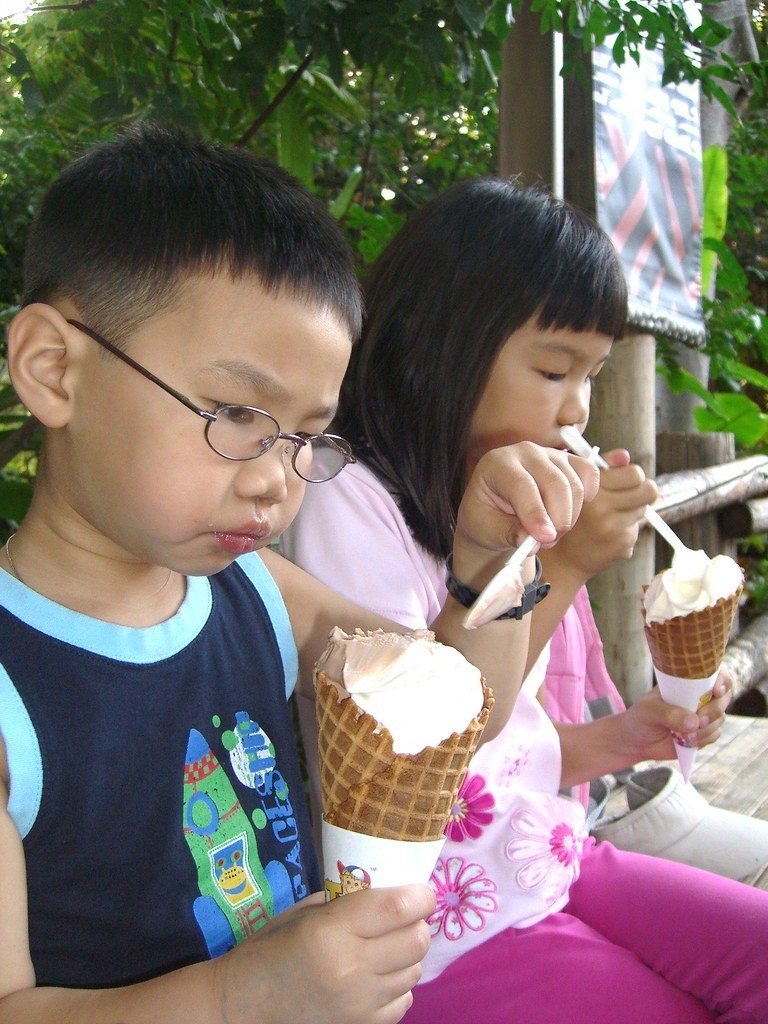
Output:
<box><xmin>445</xmin><ymin>551</ymin><xmax>551</xmax><ymax>621</ymax></box>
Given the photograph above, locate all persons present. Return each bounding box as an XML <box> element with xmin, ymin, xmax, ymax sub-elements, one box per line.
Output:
<box><xmin>0</xmin><ymin>120</ymin><xmax>600</xmax><ymax>1023</ymax></box>
<box><xmin>279</xmin><ymin>179</ymin><xmax>768</xmax><ymax>1024</ymax></box>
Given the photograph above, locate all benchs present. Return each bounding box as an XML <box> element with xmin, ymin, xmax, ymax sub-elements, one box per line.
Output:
<box><xmin>591</xmin><ymin>710</ymin><xmax>768</xmax><ymax>902</ymax></box>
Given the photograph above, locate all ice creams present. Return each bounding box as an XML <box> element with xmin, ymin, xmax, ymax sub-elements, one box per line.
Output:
<box><xmin>310</xmin><ymin>627</ymin><xmax>494</xmax><ymax>843</ymax></box>
<box><xmin>640</xmin><ymin>547</ymin><xmax>746</xmax><ymax>678</ymax></box>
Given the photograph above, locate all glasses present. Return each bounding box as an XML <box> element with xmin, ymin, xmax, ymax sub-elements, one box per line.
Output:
<box><xmin>66</xmin><ymin>319</ymin><xmax>357</xmax><ymax>483</ymax></box>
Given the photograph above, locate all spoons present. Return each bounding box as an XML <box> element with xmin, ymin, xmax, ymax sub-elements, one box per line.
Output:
<box><xmin>561</xmin><ymin>423</ymin><xmax>707</xmax><ymax>581</ymax></box>
<box><xmin>462</xmin><ymin>534</ymin><xmax>540</xmax><ymax>630</ymax></box>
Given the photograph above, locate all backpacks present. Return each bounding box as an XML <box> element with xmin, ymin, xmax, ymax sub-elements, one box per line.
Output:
<box><xmin>538</xmin><ymin>584</ymin><xmax>628</xmax><ymax>814</ymax></box>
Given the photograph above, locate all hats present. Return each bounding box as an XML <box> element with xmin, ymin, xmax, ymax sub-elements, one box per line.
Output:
<box><xmin>592</xmin><ymin>766</ymin><xmax>768</xmax><ymax>880</ymax></box>
